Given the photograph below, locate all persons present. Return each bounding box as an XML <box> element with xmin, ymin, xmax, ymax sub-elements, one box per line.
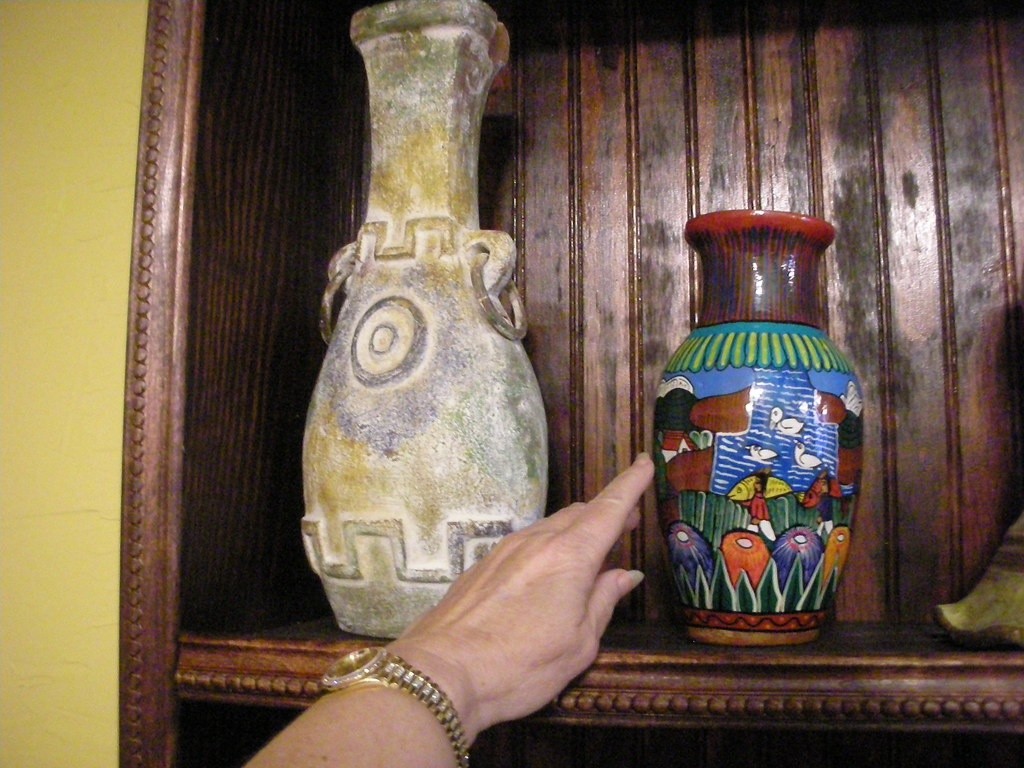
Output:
<box><xmin>239</xmin><ymin>451</ymin><xmax>656</xmax><ymax>768</ymax></box>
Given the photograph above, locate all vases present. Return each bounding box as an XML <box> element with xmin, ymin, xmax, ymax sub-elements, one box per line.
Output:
<box><xmin>301</xmin><ymin>0</ymin><xmax>548</xmax><ymax>639</ymax></box>
<box><xmin>652</xmin><ymin>209</ymin><xmax>864</xmax><ymax>647</ymax></box>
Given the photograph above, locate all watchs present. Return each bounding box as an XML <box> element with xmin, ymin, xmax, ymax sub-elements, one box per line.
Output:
<box><xmin>320</xmin><ymin>646</ymin><xmax>471</xmax><ymax>768</ymax></box>
